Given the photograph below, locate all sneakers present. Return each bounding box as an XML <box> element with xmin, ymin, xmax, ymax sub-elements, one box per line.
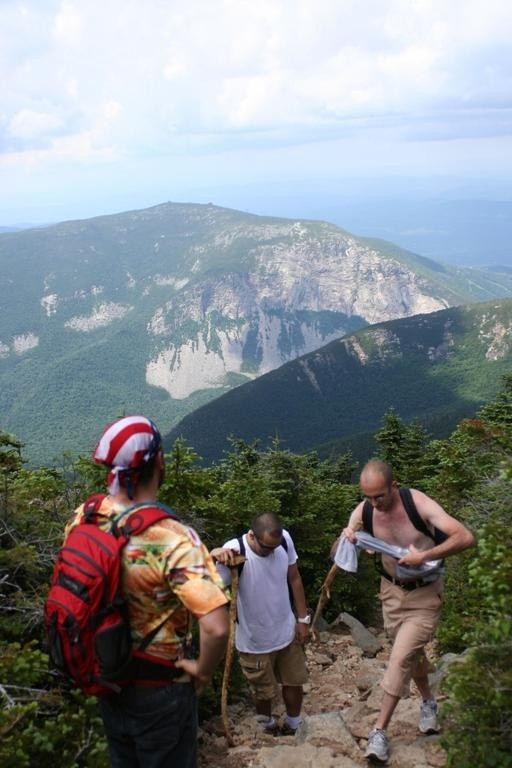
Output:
<box><xmin>364</xmin><ymin>728</ymin><xmax>391</xmax><ymax>762</ymax></box>
<box><xmin>418</xmin><ymin>698</ymin><xmax>442</xmax><ymax>734</ymax></box>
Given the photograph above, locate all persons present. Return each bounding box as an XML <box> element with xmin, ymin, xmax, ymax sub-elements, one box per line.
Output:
<box><xmin>329</xmin><ymin>457</ymin><xmax>478</xmax><ymax>766</ymax></box>
<box><xmin>61</xmin><ymin>412</ymin><xmax>234</xmax><ymax>768</ymax></box>
<box><xmin>208</xmin><ymin>511</ymin><xmax>314</xmax><ymax>738</ymax></box>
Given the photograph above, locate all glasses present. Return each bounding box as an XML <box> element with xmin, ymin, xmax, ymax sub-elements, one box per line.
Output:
<box><xmin>255</xmin><ymin>536</ymin><xmax>276</xmax><ymax>550</ymax></box>
<box><xmin>360</xmin><ymin>494</ymin><xmax>384</xmax><ymax>503</ymax></box>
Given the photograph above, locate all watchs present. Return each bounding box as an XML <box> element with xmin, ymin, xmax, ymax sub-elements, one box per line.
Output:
<box><xmin>296</xmin><ymin>616</ymin><xmax>313</xmax><ymax>626</ymax></box>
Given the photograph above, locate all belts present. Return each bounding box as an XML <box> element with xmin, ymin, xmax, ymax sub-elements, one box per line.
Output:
<box><xmin>382</xmin><ymin>571</ymin><xmax>423</xmax><ymax>591</ymax></box>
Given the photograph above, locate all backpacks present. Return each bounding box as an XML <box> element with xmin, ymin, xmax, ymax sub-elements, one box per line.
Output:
<box><xmin>362</xmin><ymin>488</ymin><xmax>450</xmax><ymax>586</ymax></box>
<box><xmin>44</xmin><ymin>492</ymin><xmax>180</xmax><ymax>699</ymax></box>
